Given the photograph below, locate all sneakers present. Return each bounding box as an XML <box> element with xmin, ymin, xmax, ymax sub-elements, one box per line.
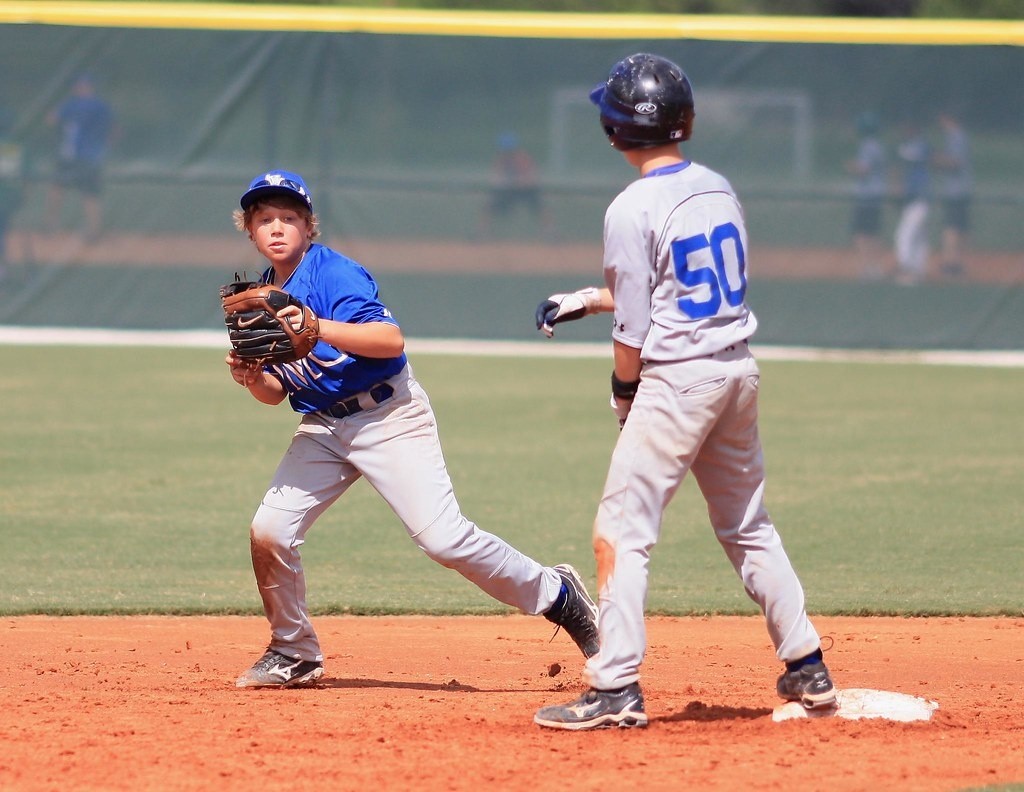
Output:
<box><xmin>775</xmin><ymin>647</ymin><xmax>837</xmax><ymax>709</ymax></box>
<box><xmin>542</xmin><ymin>563</ymin><xmax>601</xmax><ymax>660</ymax></box>
<box><xmin>235</xmin><ymin>647</ymin><xmax>327</xmax><ymax>690</ymax></box>
<box><xmin>533</xmin><ymin>680</ymin><xmax>648</xmax><ymax>730</ymax></box>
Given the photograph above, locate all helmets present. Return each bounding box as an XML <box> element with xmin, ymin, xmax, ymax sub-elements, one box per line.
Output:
<box><xmin>589</xmin><ymin>52</ymin><xmax>697</xmax><ymax>145</ymax></box>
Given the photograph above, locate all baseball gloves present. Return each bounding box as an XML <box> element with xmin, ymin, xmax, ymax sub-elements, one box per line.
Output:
<box><xmin>218</xmin><ymin>281</ymin><xmax>321</xmax><ymax>366</ymax></box>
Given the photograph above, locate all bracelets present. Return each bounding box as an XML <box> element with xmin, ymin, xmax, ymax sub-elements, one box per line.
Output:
<box><xmin>611</xmin><ymin>370</ymin><xmax>641</xmax><ymax>401</ymax></box>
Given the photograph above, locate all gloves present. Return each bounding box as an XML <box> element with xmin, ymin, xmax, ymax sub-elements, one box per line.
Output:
<box><xmin>534</xmin><ymin>287</ymin><xmax>601</xmax><ymax>339</ymax></box>
<box><xmin>609</xmin><ymin>370</ymin><xmax>642</xmax><ymax>432</ymax></box>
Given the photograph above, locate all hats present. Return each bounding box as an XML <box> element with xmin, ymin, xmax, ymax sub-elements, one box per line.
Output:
<box><xmin>239</xmin><ymin>169</ymin><xmax>315</xmax><ymax>216</ymax></box>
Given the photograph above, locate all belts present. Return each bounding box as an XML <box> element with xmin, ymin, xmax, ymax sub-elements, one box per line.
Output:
<box><xmin>326</xmin><ymin>383</ymin><xmax>394</xmax><ymax>419</ymax></box>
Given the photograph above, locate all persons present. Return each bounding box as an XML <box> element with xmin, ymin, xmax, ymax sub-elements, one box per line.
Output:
<box><xmin>842</xmin><ymin>106</ymin><xmax>973</xmax><ymax>277</ymax></box>
<box><xmin>219</xmin><ymin>169</ymin><xmax>600</xmax><ymax>689</ymax></box>
<box><xmin>532</xmin><ymin>52</ymin><xmax>837</xmax><ymax>732</ymax></box>
<box><xmin>43</xmin><ymin>63</ymin><xmax>115</xmax><ymax>242</ymax></box>
